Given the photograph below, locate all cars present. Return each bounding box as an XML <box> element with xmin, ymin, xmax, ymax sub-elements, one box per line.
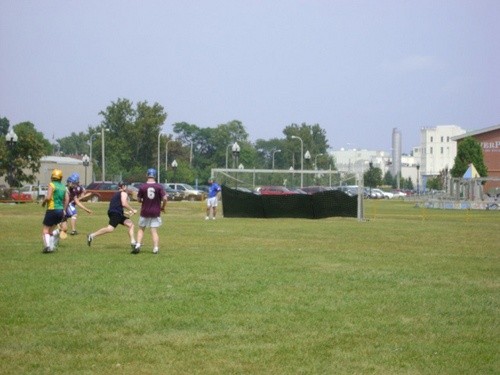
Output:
<box><xmin>11</xmin><ymin>185</ymin><xmax>48</xmax><ymax>203</ymax></box>
<box><xmin>80</xmin><ymin>181</ymin><xmax>120</xmax><ymax>202</ymax></box>
<box><xmin>303</xmin><ymin>185</ymin><xmax>407</xmax><ymax>201</ymax></box>
<box><xmin>128</xmin><ymin>182</ymin><xmax>211</xmax><ymax>202</ymax></box>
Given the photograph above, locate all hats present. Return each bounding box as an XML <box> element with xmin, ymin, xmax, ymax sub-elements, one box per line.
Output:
<box><xmin>119</xmin><ymin>180</ymin><xmax>131</xmax><ymax>185</ymax></box>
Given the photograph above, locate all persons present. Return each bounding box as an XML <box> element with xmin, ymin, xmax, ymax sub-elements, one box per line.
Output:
<box><xmin>131</xmin><ymin>169</ymin><xmax>169</xmax><ymax>255</ymax></box>
<box><xmin>86</xmin><ymin>181</ymin><xmax>137</xmax><ymax>247</ymax></box>
<box><xmin>64</xmin><ymin>173</ymin><xmax>91</xmax><ymax>234</ymax></box>
<box><xmin>204</xmin><ymin>177</ymin><xmax>222</xmax><ymax>220</ymax></box>
<box><xmin>39</xmin><ymin>169</ymin><xmax>70</xmax><ymax>253</ymax></box>
<box><xmin>65</xmin><ymin>177</ymin><xmax>71</xmax><ymax>186</ymax></box>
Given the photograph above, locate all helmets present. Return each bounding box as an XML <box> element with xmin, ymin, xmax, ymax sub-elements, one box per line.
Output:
<box><xmin>147</xmin><ymin>168</ymin><xmax>157</xmax><ymax>178</ymax></box>
<box><xmin>67</xmin><ymin>173</ymin><xmax>79</xmax><ymax>183</ymax></box>
<box><xmin>51</xmin><ymin>169</ymin><xmax>63</xmax><ymax>181</ymax></box>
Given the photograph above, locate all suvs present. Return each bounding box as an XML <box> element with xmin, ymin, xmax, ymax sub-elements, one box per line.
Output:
<box><xmin>255</xmin><ymin>186</ymin><xmax>296</xmax><ymax>196</ymax></box>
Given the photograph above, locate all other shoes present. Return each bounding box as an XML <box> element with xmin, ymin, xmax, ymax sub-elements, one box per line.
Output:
<box><xmin>206</xmin><ymin>217</ymin><xmax>209</xmax><ymax>220</ymax></box>
<box><xmin>212</xmin><ymin>218</ymin><xmax>215</xmax><ymax>220</ymax></box>
<box><xmin>152</xmin><ymin>249</ymin><xmax>159</xmax><ymax>254</ymax></box>
<box><xmin>42</xmin><ymin>247</ymin><xmax>55</xmax><ymax>253</ymax></box>
<box><xmin>87</xmin><ymin>234</ymin><xmax>92</xmax><ymax>247</ymax></box>
<box><xmin>71</xmin><ymin>231</ymin><xmax>76</xmax><ymax>235</ymax></box>
<box><xmin>131</xmin><ymin>249</ymin><xmax>139</xmax><ymax>253</ymax></box>
<box><xmin>131</xmin><ymin>243</ymin><xmax>143</xmax><ymax>248</ymax></box>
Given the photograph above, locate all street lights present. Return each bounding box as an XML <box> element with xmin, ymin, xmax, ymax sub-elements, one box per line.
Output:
<box><xmin>90</xmin><ymin>132</ymin><xmax>101</xmax><ymax>183</ymax></box>
<box><xmin>303</xmin><ymin>149</ymin><xmax>312</xmax><ymax>194</ymax></box>
<box><xmin>101</xmin><ymin>128</ymin><xmax>110</xmax><ymax>181</ymax></box>
<box><xmin>82</xmin><ymin>153</ymin><xmax>90</xmax><ymax>189</ymax></box>
<box><xmin>165</xmin><ymin>139</ymin><xmax>176</xmax><ymax>184</ymax></box>
<box><xmin>314</xmin><ymin>153</ymin><xmax>323</xmax><ymax>169</ymax></box>
<box><xmin>272</xmin><ymin>150</ymin><xmax>282</xmax><ymax>169</ymax></box>
<box><xmin>171</xmin><ymin>159</ymin><xmax>178</xmax><ymax>183</ymax></box>
<box><xmin>231</xmin><ymin>141</ymin><xmax>241</xmax><ymax>196</ymax></box>
<box><xmin>290</xmin><ymin>135</ymin><xmax>304</xmax><ymax>189</ymax></box>
